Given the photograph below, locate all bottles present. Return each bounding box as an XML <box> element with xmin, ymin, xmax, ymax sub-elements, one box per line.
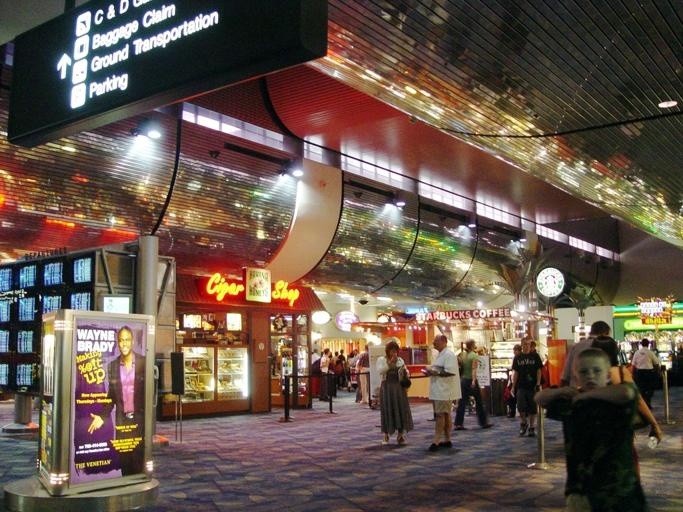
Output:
<box><xmin>648</xmin><ymin>428</ymin><xmax>665</xmax><ymax>449</ymax></box>
<box><xmin>430</xmin><ymin>366</ymin><xmax>444</xmax><ymax>375</ymax></box>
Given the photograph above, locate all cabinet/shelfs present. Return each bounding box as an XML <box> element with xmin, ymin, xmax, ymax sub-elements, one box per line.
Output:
<box><xmin>488</xmin><ymin>340</ymin><xmax>521</xmax><ymax>379</ymax></box>
<box><xmin>270</xmin><ymin>338</ymin><xmax>309</xmax><ymax>406</ymax></box>
<box><xmin>161</xmin><ymin>344</ymin><xmax>250</xmax><ymax>419</ymax></box>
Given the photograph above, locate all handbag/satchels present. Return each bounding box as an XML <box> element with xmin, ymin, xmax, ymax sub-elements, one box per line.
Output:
<box><xmin>398</xmin><ymin>365</ymin><xmax>411</xmax><ymax>388</ymax></box>
<box><xmin>632</xmin><ymin>424</ymin><xmax>658</xmax><ymax>450</ymax></box>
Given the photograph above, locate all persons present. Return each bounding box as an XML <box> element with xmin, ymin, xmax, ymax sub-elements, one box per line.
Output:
<box><xmin>312</xmin><ymin>345</ymin><xmax>369</xmax><ymax>403</ymax></box>
<box><xmin>577</xmin><ymin>335</ymin><xmax>663</xmax><ymax>445</ymax></box>
<box><xmin>651</xmin><ymin>341</ymin><xmax>659</xmax><ymax>358</ymax></box>
<box><xmin>561</xmin><ymin>321</ymin><xmax>611</xmax><ymax>389</ymax></box>
<box><xmin>423</xmin><ymin>333</ymin><xmax>462</xmax><ymax>452</ymax></box>
<box><xmin>508</xmin><ymin>336</ymin><xmax>546</xmax><ymax>436</ymax></box>
<box><xmin>376</xmin><ymin>341</ymin><xmax>414</xmax><ymax>445</ymax></box>
<box><xmin>629</xmin><ymin>341</ymin><xmax>639</xmax><ymax>359</ymax></box>
<box><xmin>454</xmin><ymin>340</ymin><xmax>495</xmax><ymax>431</ymax></box>
<box><xmin>631</xmin><ymin>339</ymin><xmax>661</xmax><ymax>408</ymax></box>
<box><xmin>88</xmin><ymin>326</ymin><xmax>146</xmax><ymax>477</ymax></box>
<box><xmin>535</xmin><ymin>348</ymin><xmax>652</xmax><ymax>512</ymax></box>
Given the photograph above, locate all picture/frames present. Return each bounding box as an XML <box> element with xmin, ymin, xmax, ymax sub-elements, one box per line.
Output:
<box><xmin>246</xmin><ymin>266</ymin><xmax>272</xmax><ymax>303</ymax></box>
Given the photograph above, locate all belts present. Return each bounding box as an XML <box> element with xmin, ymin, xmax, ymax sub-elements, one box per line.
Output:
<box><xmin>123</xmin><ymin>412</ymin><xmax>133</xmax><ymax>419</ymax></box>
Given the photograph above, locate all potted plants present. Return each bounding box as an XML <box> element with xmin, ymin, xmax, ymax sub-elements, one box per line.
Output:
<box><xmin>564</xmin><ymin>285</ymin><xmax>601</xmax><ymax>328</ymax></box>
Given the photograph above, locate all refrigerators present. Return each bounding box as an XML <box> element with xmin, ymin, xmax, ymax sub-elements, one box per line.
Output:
<box><xmin>177</xmin><ymin>344</ymin><xmax>250</xmax><ymax>416</ymax></box>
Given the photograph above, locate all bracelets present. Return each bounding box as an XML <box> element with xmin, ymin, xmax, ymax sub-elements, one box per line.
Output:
<box><xmin>652</xmin><ymin>423</ymin><xmax>658</xmax><ymax>426</ymax></box>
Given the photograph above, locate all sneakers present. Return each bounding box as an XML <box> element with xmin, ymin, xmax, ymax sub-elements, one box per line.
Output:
<box><xmin>396</xmin><ymin>435</ymin><xmax>408</xmax><ymax>445</ymax></box>
<box><xmin>519</xmin><ymin>423</ymin><xmax>528</xmax><ymax>434</ymax></box>
<box><xmin>527</xmin><ymin>427</ymin><xmax>534</xmax><ymax>437</ymax></box>
<box><xmin>382</xmin><ymin>436</ymin><xmax>389</xmax><ymax>445</ymax></box>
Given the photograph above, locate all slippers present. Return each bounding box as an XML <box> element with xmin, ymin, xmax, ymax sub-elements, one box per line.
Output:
<box><xmin>454</xmin><ymin>426</ymin><xmax>467</xmax><ymax>430</ymax></box>
<box><xmin>482</xmin><ymin>423</ymin><xmax>493</xmax><ymax>428</ymax></box>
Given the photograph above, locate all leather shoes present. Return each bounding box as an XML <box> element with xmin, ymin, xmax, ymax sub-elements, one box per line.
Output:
<box><xmin>441</xmin><ymin>440</ymin><xmax>452</xmax><ymax>447</ymax></box>
<box><xmin>428</xmin><ymin>442</ymin><xmax>441</xmax><ymax>451</ymax></box>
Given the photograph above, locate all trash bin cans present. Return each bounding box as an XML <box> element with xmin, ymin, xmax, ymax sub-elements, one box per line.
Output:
<box><xmin>15</xmin><ymin>385</ymin><xmax>31</xmax><ymax>424</ymax></box>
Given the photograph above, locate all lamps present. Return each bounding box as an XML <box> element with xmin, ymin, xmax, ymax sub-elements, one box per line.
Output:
<box><xmin>392</xmin><ymin>197</ymin><xmax>405</xmax><ymax>207</ymax></box>
<box><xmin>137</xmin><ymin>120</ymin><xmax>164</xmax><ymax>139</ymax></box>
<box><xmin>279</xmin><ymin>158</ymin><xmax>304</xmax><ymax>177</ymax></box>
<box><xmin>463</xmin><ymin>215</ymin><xmax>477</xmax><ymax>228</ymax></box>
